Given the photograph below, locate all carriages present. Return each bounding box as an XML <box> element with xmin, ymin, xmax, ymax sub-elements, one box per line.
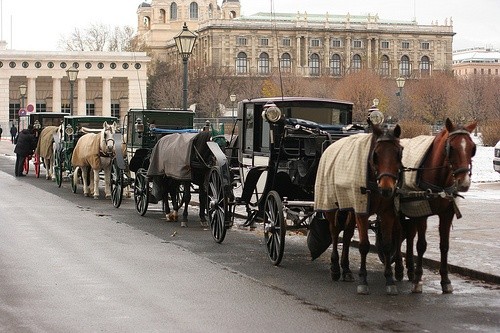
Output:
<box><xmin>53</xmin><ymin>114</ymin><xmax>118</xmax><ymax>200</ymax></box>
<box><xmin>203</xmin><ymin>97</ymin><xmax>480</xmax><ymax>295</ymax></box>
<box><xmin>14</xmin><ymin>113</ymin><xmax>71</xmax><ymax>181</ymax></box>
<box><xmin>122</xmin><ymin>108</ymin><xmax>230</xmax><ymax>228</ymax></box>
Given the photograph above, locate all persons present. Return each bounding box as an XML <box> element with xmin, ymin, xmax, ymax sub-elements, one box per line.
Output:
<box><xmin>14</xmin><ymin>124</ymin><xmax>35</xmax><ymax>177</ymax></box>
<box><xmin>10</xmin><ymin>124</ymin><xmax>17</xmax><ymax>144</ymax></box>
<box><xmin>0</xmin><ymin>124</ymin><xmax>2</xmax><ymax>138</ymax></box>
<box><xmin>128</xmin><ymin>142</ymin><xmax>154</xmax><ymax>174</ymax></box>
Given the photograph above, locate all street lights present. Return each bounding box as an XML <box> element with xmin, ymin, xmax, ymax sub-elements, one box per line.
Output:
<box><xmin>395</xmin><ymin>78</ymin><xmax>406</xmax><ymax>121</ymax></box>
<box><xmin>19</xmin><ymin>84</ymin><xmax>28</xmax><ymax>107</ymax></box>
<box><xmin>173</xmin><ymin>21</ymin><xmax>198</xmax><ymax>111</ymax></box>
<box><xmin>66</xmin><ymin>66</ymin><xmax>79</xmax><ymax>115</ymax></box>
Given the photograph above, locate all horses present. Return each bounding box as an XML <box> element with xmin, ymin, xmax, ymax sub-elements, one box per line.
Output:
<box><xmin>315</xmin><ymin>115</ymin><xmax>478</xmax><ymax>296</ymax></box>
<box><xmin>150</xmin><ymin>122</ymin><xmax>234</xmax><ymax>229</ymax></box>
<box><xmin>71</xmin><ymin>120</ymin><xmax>132</xmax><ymax>199</ymax></box>
<box><xmin>40</xmin><ymin>121</ymin><xmax>74</xmax><ymax>182</ymax></box>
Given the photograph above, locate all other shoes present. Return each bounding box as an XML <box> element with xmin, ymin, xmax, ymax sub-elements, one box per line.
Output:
<box><xmin>16</xmin><ymin>175</ymin><xmax>26</xmax><ymax>177</ymax></box>
